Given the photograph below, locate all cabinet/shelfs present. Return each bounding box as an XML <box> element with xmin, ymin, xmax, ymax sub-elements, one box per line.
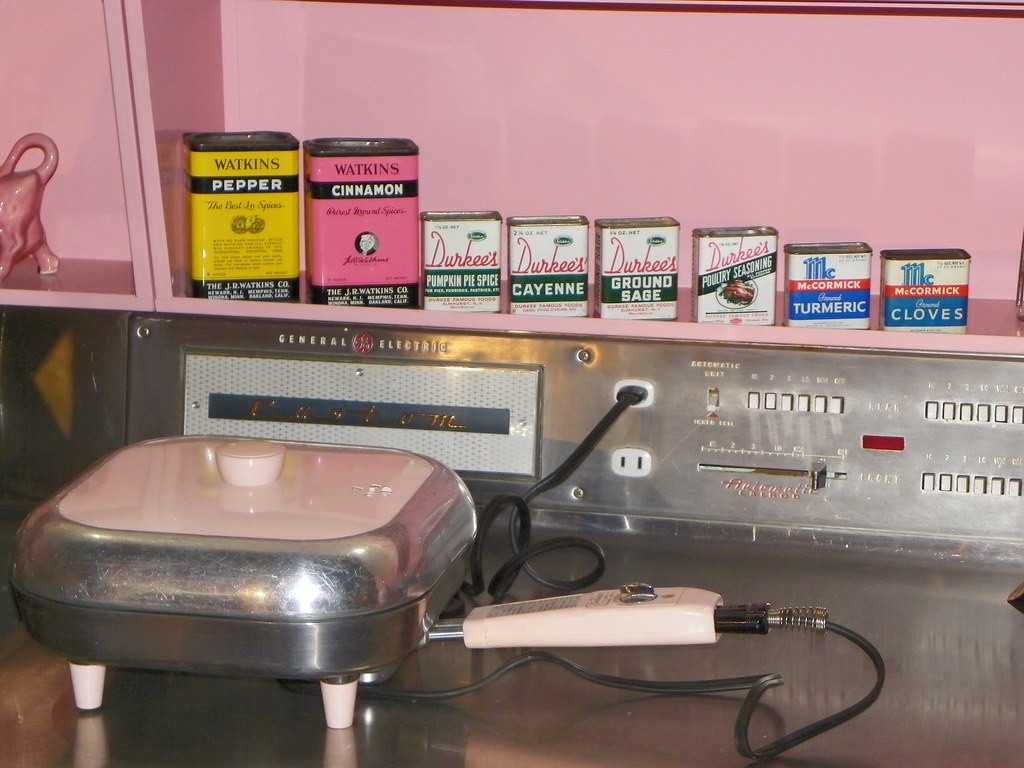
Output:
<box><xmin>0</xmin><ymin>0</ymin><xmax>1024</xmax><ymax>369</ymax></box>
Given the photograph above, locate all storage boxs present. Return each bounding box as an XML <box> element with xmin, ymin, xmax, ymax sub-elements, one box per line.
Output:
<box><xmin>506</xmin><ymin>215</ymin><xmax>591</xmax><ymax>321</ymax></box>
<box><xmin>782</xmin><ymin>241</ymin><xmax>873</xmax><ymax>331</ymax></box>
<box><xmin>183</xmin><ymin>129</ymin><xmax>301</xmax><ymax>305</ymax></box>
<box><xmin>595</xmin><ymin>217</ymin><xmax>681</xmax><ymax>322</ymax></box>
<box><xmin>302</xmin><ymin>138</ymin><xmax>422</xmax><ymax>311</ymax></box>
<box><xmin>879</xmin><ymin>248</ymin><xmax>970</xmax><ymax>334</ymax></box>
<box><xmin>690</xmin><ymin>223</ymin><xmax>779</xmax><ymax>326</ymax></box>
<box><xmin>419</xmin><ymin>209</ymin><xmax>504</xmax><ymax>315</ymax></box>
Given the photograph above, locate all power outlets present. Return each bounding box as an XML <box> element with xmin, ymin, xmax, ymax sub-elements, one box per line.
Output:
<box><xmin>610</xmin><ymin>446</ymin><xmax>651</xmax><ymax>479</ymax></box>
<box><xmin>615</xmin><ymin>380</ymin><xmax>656</xmax><ymax>410</ymax></box>
